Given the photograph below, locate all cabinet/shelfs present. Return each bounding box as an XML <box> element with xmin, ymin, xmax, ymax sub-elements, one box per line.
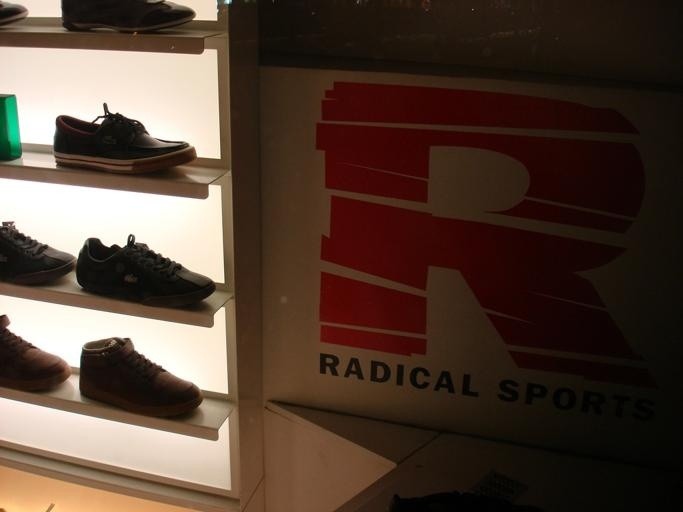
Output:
<box><xmin>0</xmin><ymin>15</ymin><xmax>262</xmax><ymax>512</ymax></box>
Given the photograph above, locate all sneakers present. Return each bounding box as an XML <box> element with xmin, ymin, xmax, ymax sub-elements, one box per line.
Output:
<box><xmin>53</xmin><ymin>104</ymin><xmax>196</xmax><ymax>176</ymax></box>
<box><xmin>0</xmin><ymin>314</ymin><xmax>70</xmax><ymax>392</ymax></box>
<box><xmin>60</xmin><ymin>0</ymin><xmax>196</xmax><ymax>33</ymax></box>
<box><xmin>78</xmin><ymin>337</ymin><xmax>202</xmax><ymax>417</ymax></box>
<box><xmin>75</xmin><ymin>234</ymin><xmax>216</xmax><ymax>308</ymax></box>
<box><xmin>0</xmin><ymin>221</ymin><xmax>77</xmax><ymax>286</ymax></box>
<box><xmin>0</xmin><ymin>0</ymin><xmax>28</xmax><ymax>24</ymax></box>
